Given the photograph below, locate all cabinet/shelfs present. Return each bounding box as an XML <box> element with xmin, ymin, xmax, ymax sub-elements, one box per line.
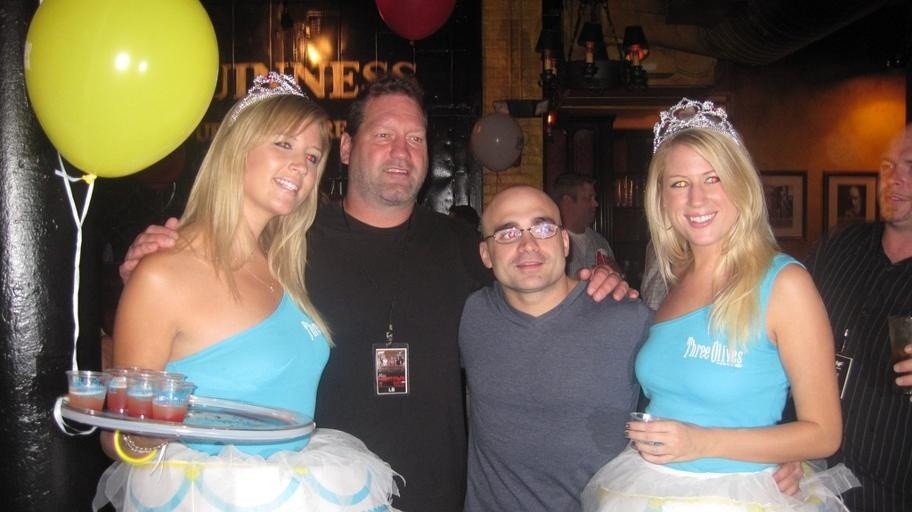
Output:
<box><xmin>612</xmin><ymin>123</ymin><xmax>657</xmax><ymax>288</ymax></box>
<box><xmin>547</xmin><ymin>113</ymin><xmax>615</xmax><ymax>262</ymax></box>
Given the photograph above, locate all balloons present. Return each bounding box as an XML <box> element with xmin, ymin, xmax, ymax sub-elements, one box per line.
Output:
<box><xmin>23</xmin><ymin>1</ymin><xmax>219</xmax><ymax>182</ymax></box>
<box><xmin>372</xmin><ymin>1</ymin><xmax>456</xmax><ymax>45</ymax></box>
<box><xmin>471</xmin><ymin>113</ymin><xmax>525</xmax><ymax>173</ymax></box>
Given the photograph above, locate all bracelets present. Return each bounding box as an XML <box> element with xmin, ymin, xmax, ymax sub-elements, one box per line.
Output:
<box><xmin>109</xmin><ymin>429</ymin><xmax>157</xmax><ymax>466</ymax></box>
<box><xmin>122</xmin><ymin>434</ymin><xmax>170</xmax><ymax>455</ymax></box>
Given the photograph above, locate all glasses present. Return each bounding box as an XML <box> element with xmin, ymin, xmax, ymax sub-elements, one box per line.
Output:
<box><xmin>482</xmin><ymin>222</ymin><xmax>564</xmax><ymax>245</ymax></box>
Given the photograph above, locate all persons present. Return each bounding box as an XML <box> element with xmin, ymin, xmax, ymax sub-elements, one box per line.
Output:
<box><xmin>785</xmin><ymin>119</ymin><xmax>912</xmax><ymax>511</ymax></box>
<box><xmin>629</xmin><ymin>125</ymin><xmax>843</xmax><ymax>512</ymax></box>
<box><xmin>447</xmin><ymin>205</ymin><xmax>481</xmax><ymax>233</ymax></box>
<box><xmin>551</xmin><ymin>171</ymin><xmax>624</xmax><ymax>290</ymax></box>
<box><xmin>118</xmin><ymin>76</ymin><xmax>645</xmax><ymax>511</ymax></box>
<box><xmin>100</xmin><ymin>91</ymin><xmax>331</xmax><ymax>511</ymax></box>
<box><xmin>456</xmin><ymin>184</ymin><xmax>804</xmax><ymax>512</ymax></box>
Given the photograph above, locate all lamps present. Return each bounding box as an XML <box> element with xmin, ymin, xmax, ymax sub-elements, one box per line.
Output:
<box><xmin>527</xmin><ymin>0</ymin><xmax>660</xmax><ymax>94</ymax></box>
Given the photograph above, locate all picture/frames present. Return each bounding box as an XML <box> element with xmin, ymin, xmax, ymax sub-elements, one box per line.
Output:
<box><xmin>817</xmin><ymin>167</ymin><xmax>882</xmax><ymax>239</ymax></box>
<box><xmin>751</xmin><ymin>167</ymin><xmax>808</xmax><ymax>244</ymax></box>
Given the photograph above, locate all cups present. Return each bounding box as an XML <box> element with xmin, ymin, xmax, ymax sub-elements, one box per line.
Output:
<box><xmin>886</xmin><ymin>316</ymin><xmax>912</xmax><ymax>396</ymax></box>
<box><xmin>65</xmin><ymin>366</ymin><xmax>198</xmax><ymax>425</ymax></box>
<box><xmin>629</xmin><ymin>411</ymin><xmax>657</xmax><ymax>453</ymax></box>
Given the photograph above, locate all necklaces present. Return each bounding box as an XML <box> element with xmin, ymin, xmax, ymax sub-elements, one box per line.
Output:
<box><xmin>228</xmin><ymin>247</ymin><xmax>280</xmax><ymax>300</ymax></box>
